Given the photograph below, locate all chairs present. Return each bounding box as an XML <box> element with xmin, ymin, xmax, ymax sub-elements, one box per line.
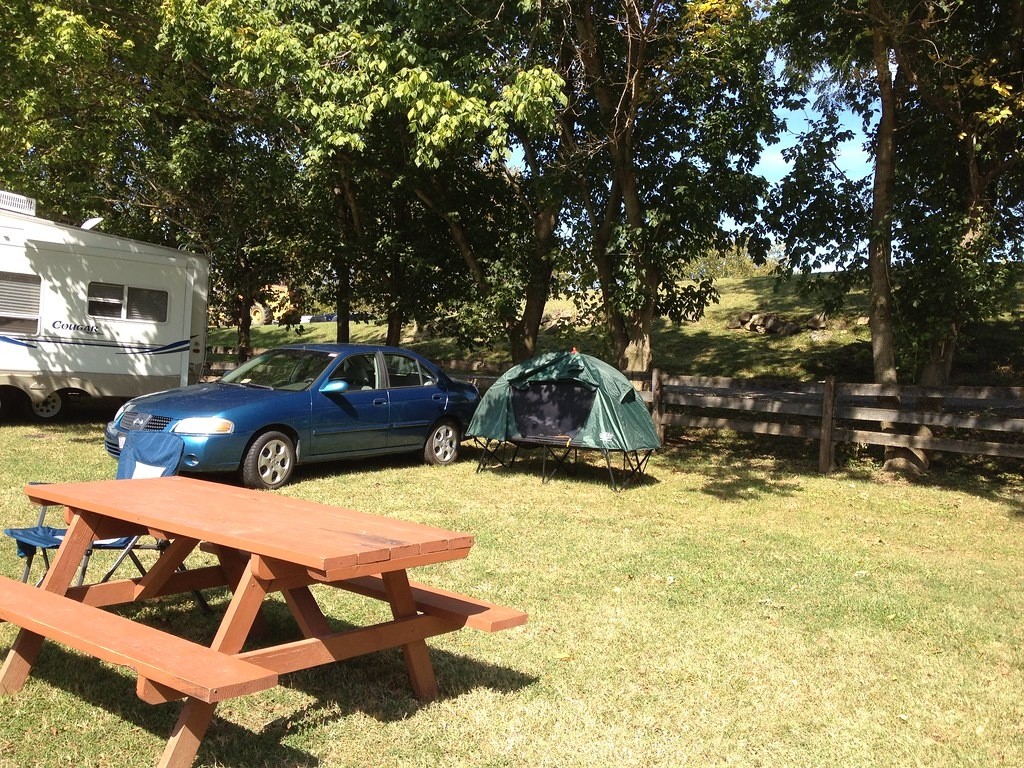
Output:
<box><xmin>345</xmin><ymin>366</ymin><xmax>373</xmax><ymax>390</ymax></box>
<box><xmin>5</xmin><ymin>430</ymin><xmax>212</xmax><ymax>616</ymax></box>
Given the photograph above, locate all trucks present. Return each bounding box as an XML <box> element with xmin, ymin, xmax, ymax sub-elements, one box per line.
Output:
<box><xmin>0</xmin><ymin>187</ymin><xmax>215</xmax><ymax>425</ymax></box>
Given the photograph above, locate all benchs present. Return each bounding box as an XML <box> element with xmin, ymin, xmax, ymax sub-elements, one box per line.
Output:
<box><xmin>1</xmin><ymin>541</ymin><xmax>528</xmax><ymax>703</ymax></box>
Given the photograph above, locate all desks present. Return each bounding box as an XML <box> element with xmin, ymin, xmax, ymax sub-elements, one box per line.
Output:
<box><xmin>0</xmin><ymin>476</ymin><xmax>475</xmax><ymax>768</ymax></box>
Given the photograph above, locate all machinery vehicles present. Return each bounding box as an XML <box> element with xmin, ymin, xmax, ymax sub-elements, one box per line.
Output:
<box><xmin>209</xmin><ymin>282</ymin><xmax>298</xmax><ymax>327</ymax></box>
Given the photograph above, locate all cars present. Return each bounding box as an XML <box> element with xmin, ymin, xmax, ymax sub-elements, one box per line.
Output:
<box><xmin>103</xmin><ymin>339</ymin><xmax>483</xmax><ymax>493</ymax></box>
<box><xmin>323</xmin><ymin>310</ymin><xmax>377</xmax><ymax>323</ymax></box>
